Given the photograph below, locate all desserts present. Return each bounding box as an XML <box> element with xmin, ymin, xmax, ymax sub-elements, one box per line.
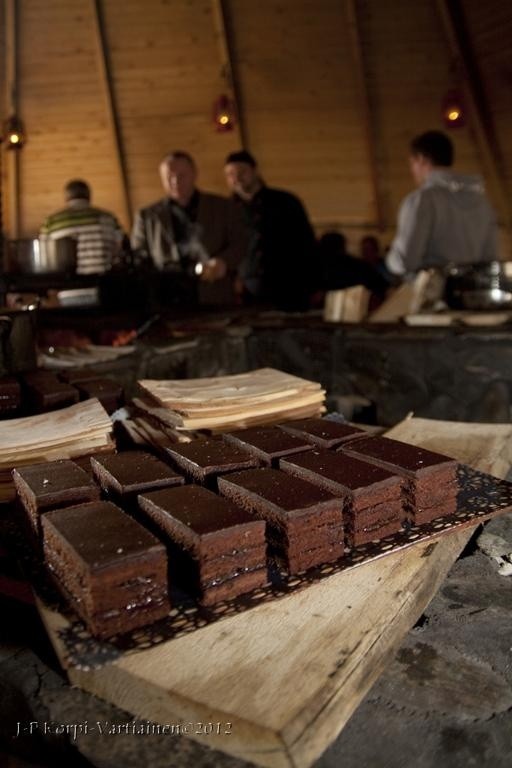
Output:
<box><xmin>10</xmin><ymin>416</ymin><xmax>460</xmax><ymax>637</ymax></box>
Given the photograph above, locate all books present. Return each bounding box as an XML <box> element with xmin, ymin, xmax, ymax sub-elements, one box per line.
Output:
<box><xmin>1</xmin><ymin>396</ymin><xmax>114</xmax><ymax>464</ymax></box>
<box><xmin>130</xmin><ymin>366</ymin><xmax>326</xmax><ymax>433</ymax></box>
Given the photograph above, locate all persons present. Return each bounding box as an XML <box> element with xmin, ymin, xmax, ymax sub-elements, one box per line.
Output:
<box><xmin>383</xmin><ymin>126</ymin><xmax>498</xmax><ymax>310</ymax></box>
<box><xmin>221</xmin><ymin>150</ymin><xmax>329</xmax><ymax>310</ymax></box>
<box><xmin>128</xmin><ymin>149</ymin><xmax>236</xmax><ymax>311</ymax></box>
<box><xmin>317</xmin><ymin>231</ymin><xmax>379</xmax><ymax>301</ymax></box>
<box><xmin>358</xmin><ymin>235</ymin><xmax>383</xmax><ymax>266</ymax></box>
<box><xmin>39</xmin><ymin>179</ymin><xmax>135</xmax><ymax>277</ymax></box>
